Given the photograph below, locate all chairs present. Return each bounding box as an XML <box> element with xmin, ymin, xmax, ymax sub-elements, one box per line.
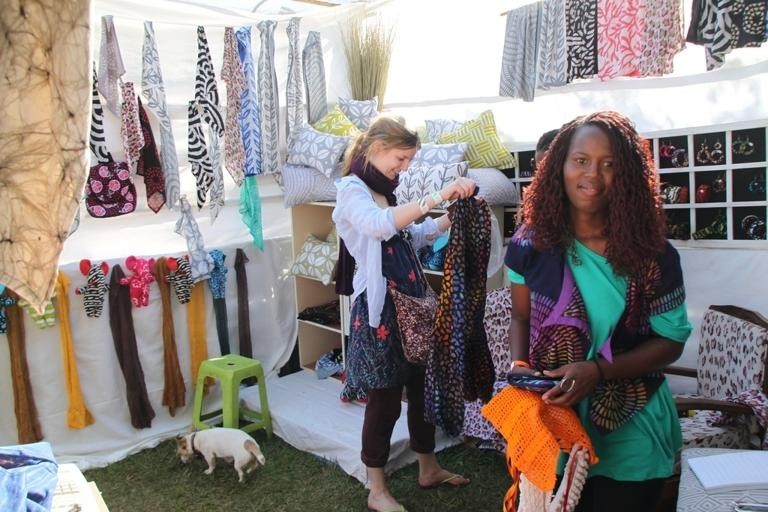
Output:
<box><xmin>661</xmin><ymin>303</ymin><xmax>768</xmax><ymax>488</ymax></box>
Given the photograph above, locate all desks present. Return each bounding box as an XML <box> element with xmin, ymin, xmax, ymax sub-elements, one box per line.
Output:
<box><xmin>676</xmin><ymin>447</ymin><xmax>768</xmax><ymax>512</ymax></box>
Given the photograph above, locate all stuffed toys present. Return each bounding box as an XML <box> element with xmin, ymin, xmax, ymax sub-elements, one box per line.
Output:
<box><xmin>74</xmin><ymin>258</ymin><xmax>113</xmax><ymax>318</ymax></box>
<box><xmin>1</xmin><ymin>295</ymin><xmax>17</xmax><ymax>334</ymax></box>
<box><xmin>119</xmin><ymin>255</ymin><xmax>156</xmax><ymax>308</ymax></box>
<box><xmin>17</xmin><ymin>291</ymin><xmax>59</xmax><ymax>330</ymax></box>
<box><xmin>160</xmin><ymin>255</ymin><xmax>194</xmax><ymax>304</ymax></box>
<box><xmin>206</xmin><ymin>249</ymin><xmax>228</xmax><ymax>300</ymax></box>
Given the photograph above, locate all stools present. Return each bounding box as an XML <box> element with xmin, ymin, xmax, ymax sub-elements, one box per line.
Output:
<box><xmin>191</xmin><ymin>354</ymin><xmax>273</xmax><ymax>440</ymax></box>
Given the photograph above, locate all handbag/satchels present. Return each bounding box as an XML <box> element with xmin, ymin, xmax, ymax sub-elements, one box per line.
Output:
<box><xmin>85</xmin><ymin>161</ymin><xmax>137</xmax><ymax>218</ymax></box>
<box><xmin>388</xmin><ymin>281</ymin><xmax>439</xmax><ymax>364</ymax></box>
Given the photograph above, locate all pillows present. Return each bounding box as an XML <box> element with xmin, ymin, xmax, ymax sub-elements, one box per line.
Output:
<box><xmin>278</xmin><ymin>95</ymin><xmax>521</xmax><ymax>207</ymax></box>
<box><xmin>291</xmin><ymin>234</ymin><xmax>339</xmax><ymax>285</ymax></box>
<box><xmin>325</xmin><ymin>226</ymin><xmax>338</xmax><ymax>243</ymax></box>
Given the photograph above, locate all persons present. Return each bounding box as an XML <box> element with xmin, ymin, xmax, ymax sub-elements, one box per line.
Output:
<box><xmin>533</xmin><ymin>127</ymin><xmax>562</xmax><ymax>168</ymax></box>
<box><xmin>503</xmin><ymin>108</ymin><xmax>695</xmax><ymax>511</ymax></box>
<box><xmin>329</xmin><ymin>117</ymin><xmax>473</xmax><ymax>511</ymax></box>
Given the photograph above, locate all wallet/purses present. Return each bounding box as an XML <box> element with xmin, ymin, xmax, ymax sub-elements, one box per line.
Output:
<box><xmin>508</xmin><ymin>373</ymin><xmax>562</xmax><ymax>393</ymax></box>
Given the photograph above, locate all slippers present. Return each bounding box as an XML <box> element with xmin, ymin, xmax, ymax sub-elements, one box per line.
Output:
<box><xmin>372</xmin><ymin>504</ymin><xmax>409</xmax><ymax>512</ymax></box>
<box><xmin>427</xmin><ymin>471</ymin><xmax>470</xmax><ymax>489</ymax></box>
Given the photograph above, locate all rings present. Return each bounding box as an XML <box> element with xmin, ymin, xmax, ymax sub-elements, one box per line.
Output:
<box><xmin>559</xmin><ymin>375</ymin><xmax>577</xmax><ymax>393</ymax></box>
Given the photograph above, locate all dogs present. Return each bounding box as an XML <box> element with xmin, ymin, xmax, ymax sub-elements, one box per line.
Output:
<box><xmin>175</xmin><ymin>426</ymin><xmax>266</xmax><ymax>484</ymax></box>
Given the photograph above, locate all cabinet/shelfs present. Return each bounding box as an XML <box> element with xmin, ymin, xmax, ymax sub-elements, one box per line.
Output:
<box><xmin>290</xmin><ymin>194</ymin><xmax>505</xmax><ymax>403</ymax></box>
<box><xmin>501</xmin><ymin>118</ymin><xmax>768</xmax><ymax>249</ymax></box>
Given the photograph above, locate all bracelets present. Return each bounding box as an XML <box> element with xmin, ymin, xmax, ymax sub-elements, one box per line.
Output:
<box><xmin>431</xmin><ymin>192</ymin><xmax>442</xmax><ymax>206</ymax></box>
<box><xmin>510</xmin><ymin>360</ymin><xmax>530</xmax><ymax>371</ymax></box>
<box><xmin>415</xmin><ymin>198</ymin><xmax>429</xmax><ymax>216</ymax></box>
